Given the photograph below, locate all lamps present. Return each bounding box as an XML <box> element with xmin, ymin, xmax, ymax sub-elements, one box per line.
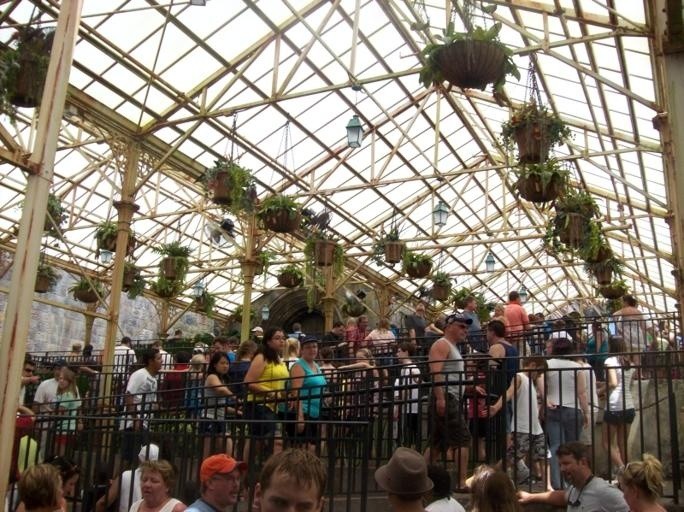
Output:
<box><xmin>433</xmin><ymin>175</ymin><xmax>449</xmax><ymax>225</ymax></box>
<box><xmin>486</xmin><ymin>231</ymin><xmax>495</xmax><ymax>275</ymax></box>
<box><xmin>346</xmin><ymin>85</ymin><xmax>365</xmax><ymax>150</ymax></box>
<box><xmin>518</xmin><ymin>268</ymin><xmax>528</xmax><ymax>303</ymax></box>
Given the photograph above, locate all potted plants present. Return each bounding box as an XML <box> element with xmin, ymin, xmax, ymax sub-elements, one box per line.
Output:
<box><xmin>200</xmin><ymin>113</ymin><xmax>257</xmax><ymax>214</ymax></box>
<box><xmin>410</xmin><ymin>0</ymin><xmax>520</xmax><ymax>94</ymax></box>
<box><xmin>371</xmin><ymin>208</ymin><xmax>405</xmax><ymax>266</ymax></box>
<box><xmin>34</xmin><ymin>191</ymin><xmax>271</xmax><ymax>322</ymax></box>
<box><xmin>431</xmin><ymin>250</ymin><xmax>457</xmax><ymax>301</ymax></box>
<box><xmin>305</xmin><ymin>201</ymin><xmax>343</xmax><ymax>311</ymax></box>
<box><xmin>453</xmin><ymin>278</ymin><xmax>481</xmax><ymax>310</ymax></box>
<box><xmin>277</xmin><ymin>254</ymin><xmax>304</xmax><ymax>288</ymax></box>
<box><xmin>255</xmin><ymin>124</ymin><xmax>303</xmax><ymax>233</ymax></box>
<box><xmin>403</xmin><ymin>230</ymin><xmax>433</xmax><ymax>280</ymax></box>
<box><xmin>0</xmin><ymin>0</ymin><xmax>57</xmax><ymax>121</ymax></box>
<box><xmin>494</xmin><ymin>56</ymin><xmax>632</xmax><ymax>299</ymax></box>
<box><xmin>476</xmin><ymin>295</ymin><xmax>503</xmax><ymax>323</ymax></box>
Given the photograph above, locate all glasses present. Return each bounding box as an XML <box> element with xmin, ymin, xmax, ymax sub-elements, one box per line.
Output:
<box><xmin>567</xmin><ymin>486</ymin><xmax>584</xmax><ymax>507</ymax></box>
<box><xmin>271</xmin><ymin>336</ymin><xmax>287</xmax><ymax>341</ymax></box>
<box><xmin>453</xmin><ymin>324</ymin><xmax>470</xmax><ymax>330</ymax></box>
<box><xmin>24</xmin><ymin>368</ymin><xmax>35</xmax><ymax>372</ymax></box>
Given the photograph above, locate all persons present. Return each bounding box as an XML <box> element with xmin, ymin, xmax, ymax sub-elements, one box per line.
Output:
<box><xmin>1</xmin><ymin>291</ymin><xmax>684</xmax><ymax>512</ymax></box>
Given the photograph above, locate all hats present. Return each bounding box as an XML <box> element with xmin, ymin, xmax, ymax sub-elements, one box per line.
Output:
<box><xmin>442</xmin><ymin>314</ymin><xmax>473</xmax><ymax>329</ymax></box>
<box><xmin>251</xmin><ymin>326</ymin><xmax>263</xmax><ymax>333</ymax></box>
<box><xmin>374</xmin><ymin>446</ymin><xmax>435</xmax><ymax>495</ymax></box>
<box><xmin>138</xmin><ymin>443</ymin><xmax>160</xmax><ymax>461</ymax></box>
<box><xmin>199</xmin><ymin>454</ymin><xmax>249</xmax><ymax>480</ymax></box>
<box><xmin>301</xmin><ymin>334</ymin><xmax>319</xmax><ymax>345</ymax></box>
<box><xmin>53</xmin><ymin>359</ymin><xmax>67</xmax><ymax>367</ymax></box>
<box><xmin>292</xmin><ymin>323</ymin><xmax>301</xmax><ymax>331</ymax></box>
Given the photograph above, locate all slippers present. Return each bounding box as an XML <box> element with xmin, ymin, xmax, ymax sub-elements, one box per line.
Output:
<box><xmin>454</xmin><ymin>484</ymin><xmax>473</xmax><ymax>493</ymax></box>
